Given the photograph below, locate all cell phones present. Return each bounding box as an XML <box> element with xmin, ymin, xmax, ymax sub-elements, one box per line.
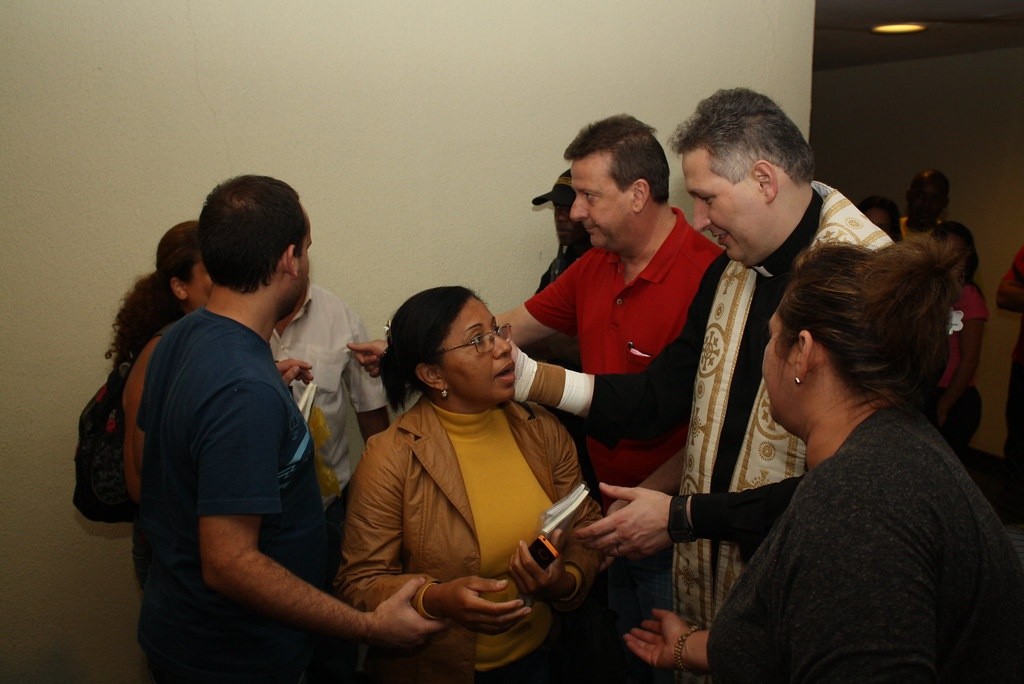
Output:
<box><xmin>529</xmin><ymin>536</ymin><xmax>558</xmax><ymax>570</ymax></box>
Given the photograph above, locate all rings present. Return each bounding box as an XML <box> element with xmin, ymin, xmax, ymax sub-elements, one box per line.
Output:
<box><xmin>617</xmin><ymin>544</ymin><xmax>621</xmax><ymax>553</ymax></box>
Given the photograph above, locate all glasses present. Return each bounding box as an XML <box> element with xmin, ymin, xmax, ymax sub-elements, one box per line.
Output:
<box><xmin>423</xmin><ymin>321</ymin><xmax>512</xmax><ymax>362</ymax></box>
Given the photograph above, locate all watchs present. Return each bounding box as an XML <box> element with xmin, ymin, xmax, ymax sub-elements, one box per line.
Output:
<box><xmin>667</xmin><ymin>494</ymin><xmax>697</xmax><ymax>544</ymax></box>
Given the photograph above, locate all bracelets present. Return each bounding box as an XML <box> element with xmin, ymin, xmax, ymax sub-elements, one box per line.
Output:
<box><xmin>674</xmin><ymin>625</ymin><xmax>700</xmax><ymax>672</ymax></box>
<box><xmin>412</xmin><ymin>581</ymin><xmax>440</xmax><ymax>620</ymax></box>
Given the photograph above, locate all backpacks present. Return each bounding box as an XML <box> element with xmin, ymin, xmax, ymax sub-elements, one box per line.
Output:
<box><xmin>72</xmin><ymin>308</ymin><xmax>186</xmax><ymax>524</ymax></box>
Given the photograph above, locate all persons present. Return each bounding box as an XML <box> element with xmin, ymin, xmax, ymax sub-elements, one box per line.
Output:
<box><xmin>531</xmin><ymin>169</ymin><xmax>592</xmax><ymax>509</ymax></box>
<box><xmin>505</xmin><ymin>86</ymin><xmax>898</xmax><ymax>684</ymax></box>
<box><xmin>131</xmin><ymin>175</ymin><xmax>453</xmax><ymax>684</ymax></box>
<box><xmin>105</xmin><ymin>219</ymin><xmax>214</xmax><ymax>505</ymax></box>
<box><xmin>346</xmin><ymin>116</ymin><xmax>724</xmax><ymax>684</ymax></box>
<box><xmin>269</xmin><ymin>275</ymin><xmax>392</xmax><ymax>684</ymax></box>
<box><xmin>855</xmin><ymin>167</ymin><xmax>990</xmax><ymax>467</ymax></box>
<box><xmin>996</xmin><ymin>244</ymin><xmax>1024</xmax><ymax>523</ymax></box>
<box><xmin>334</xmin><ymin>286</ymin><xmax>604</xmax><ymax>684</ymax></box>
<box><xmin>622</xmin><ymin>234</ymin><xmax>1024</xmax><ymax>684</ymax></box>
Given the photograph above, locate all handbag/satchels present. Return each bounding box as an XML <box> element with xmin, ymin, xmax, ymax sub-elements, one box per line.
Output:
<box><xmin>551</xmin><ymin>606</ymin><xmax>626</xmax><ymax>684</ymax></box>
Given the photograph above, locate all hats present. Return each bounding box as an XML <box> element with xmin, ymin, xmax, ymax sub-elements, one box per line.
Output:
<box><xmin>532</xmin><ymin>168</ymin><xmax>576</xmax><ymax>206</ymax></box>
